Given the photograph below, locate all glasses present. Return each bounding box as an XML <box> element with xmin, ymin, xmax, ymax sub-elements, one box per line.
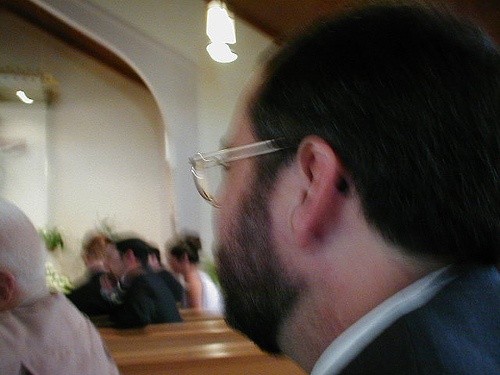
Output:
<box><xmin>189</xmin><ymin>135</ymin><xmax>352</xmax><ymax>208</ymax></box>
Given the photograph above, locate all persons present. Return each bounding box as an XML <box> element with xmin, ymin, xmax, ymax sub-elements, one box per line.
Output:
<box><xmin>67</xmin><ymin>235</ymin><xmax>118</xmax><ymax>321</ymax></box>
<box><xmin>1</xmin><ymin>195</ymin><xmax>119</xmax><ymax>375</ymax></box>
<box><xmin>188</xmin><ymin>0</ymin><xmax>500</xmax><ymax>375</ymax></box>
<box><xmin>168</xmin><ymin>234</ymin><xmax>223</xmax><ymax>312</ymax></box>
<box><xmin>146</xmin><ymin>246</ymin><xmax>184</xmax><ymax>302</ymax></box>
<box><xmin>99</xmin><ymin>239</ymin><xmax>185</xmax><ymax>329</ymax></box>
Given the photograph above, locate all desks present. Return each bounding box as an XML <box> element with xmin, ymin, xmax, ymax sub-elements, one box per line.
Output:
<box><xmin>99</xmin><ymin>319</ymin><xmax>305</xmax><ymax>375</ymax></box>
